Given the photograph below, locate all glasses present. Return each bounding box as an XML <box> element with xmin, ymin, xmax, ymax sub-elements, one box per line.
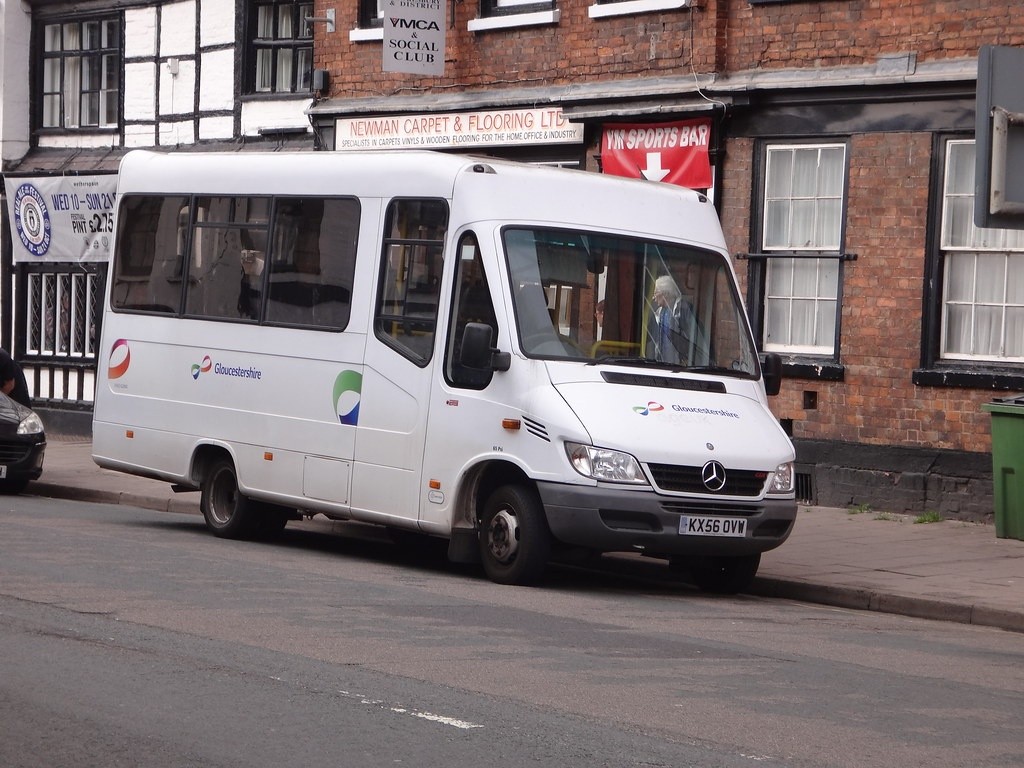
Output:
<box><xmin>594</xmin><ymin>313</ymin><xmax>601</xmax><ymax>317</ymax></box>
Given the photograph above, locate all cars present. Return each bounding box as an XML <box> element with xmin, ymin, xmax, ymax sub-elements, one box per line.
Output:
<box><xmin>0</xmin><ymin>389</ymin><xmax>47</xmax><ymax>482</ymax></box>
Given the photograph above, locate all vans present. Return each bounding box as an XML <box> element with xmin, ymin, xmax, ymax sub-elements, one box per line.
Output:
<box><xmin>90</xmin><ymin>145</ymin><xmax>798</xmax><ymax>597</ymax></box>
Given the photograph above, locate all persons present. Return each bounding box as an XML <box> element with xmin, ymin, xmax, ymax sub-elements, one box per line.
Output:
<box><xmin>645</xmin><ymin>275</ymin><xmax>706</xmax><ymax>367</ymax></box>
<box><xmin>0</xmin><ymin>347</ymin><xmax>31</xmax><ymax>409</ymax></box>
<box><xmin>595</xmin><ymin>299</ymin><xmax>606</xmax><ymax>326</ymax></box>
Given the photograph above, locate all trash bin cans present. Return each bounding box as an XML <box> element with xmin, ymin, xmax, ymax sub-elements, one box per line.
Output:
<box><xmin>980</xmin><ymin>396</ymin><xmax>1024</xmax><ymax>541</ymax></box>
